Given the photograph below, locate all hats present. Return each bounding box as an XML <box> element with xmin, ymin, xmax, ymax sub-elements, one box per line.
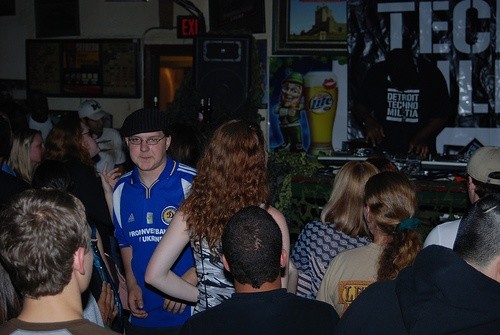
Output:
<box><xmin>466</xmin><ymin>146</ymin><xmax>500</xmax><ymax>185</ymax></box>
<box><xmin>78</xmin><ymin>100</ymin><xmax>109</xmax><ymax>121</ymax></box>
<box><xmin>120</xmin><ymin>108</ymin><xmax>169</xmax><ymax>137</ymax></box>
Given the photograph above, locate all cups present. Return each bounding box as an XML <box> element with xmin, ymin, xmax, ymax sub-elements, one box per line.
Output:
<box><xmin>305</xmin><ymin>74</ymin><xmax>338</xmax><ymax>156</ymax></box>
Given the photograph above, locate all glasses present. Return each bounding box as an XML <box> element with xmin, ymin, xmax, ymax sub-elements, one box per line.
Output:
<box><xmin>128</xmin><ymin>135</ymin><xmax>167</xmax><ymax>145</ymax></box>
<box><xmin>81</xmin><ymin>128</ymin><xmax>93</xmax><ymax>138</ymax></box>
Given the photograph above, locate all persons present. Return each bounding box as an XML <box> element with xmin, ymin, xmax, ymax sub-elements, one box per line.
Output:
<box><xmin>0</xmin><ymin>31</ymin><xmax>500</xmax><ymax>335</ymax></box>
<box><xmin>144</xmin><ymin>119</ymin><xmax>289</xmax><ymax>315</ymax></box>
